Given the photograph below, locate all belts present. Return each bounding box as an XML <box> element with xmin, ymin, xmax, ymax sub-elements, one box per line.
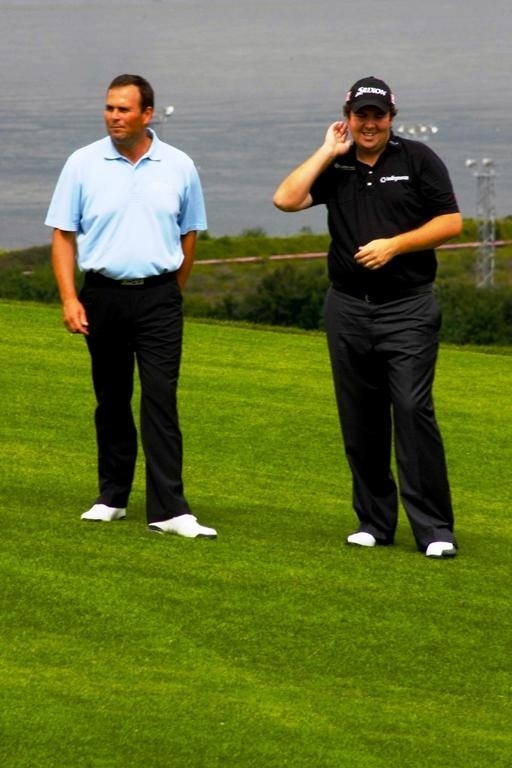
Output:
<box><xmin>330</xmin><ymin>282</ymin><xmax>433</xmax><ymax>303</ymax></box>
<box><xmin>85</xmin><ymin>272</ymin><xmax>174</xmax><ymax>287</ymax></box>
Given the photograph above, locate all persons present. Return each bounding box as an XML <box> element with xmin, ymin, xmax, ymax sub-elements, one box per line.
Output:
<box><xmin>46</xmin><ymin>74</ymin><xmax>221</xmax><ymax>541</ymax></box>
<box><xmin>270</xmin><ymin>74</ymin><xmax>465</xmax><ymax>560</ymax></box>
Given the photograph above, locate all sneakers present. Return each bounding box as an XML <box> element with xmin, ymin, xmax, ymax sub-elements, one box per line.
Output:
<box><xmin>426</xmin><ymin>541</ymin><xmax>456</xmax><ymax>557</ymax></box>
<box><xmin>347</xmin><ymin>531</ymin><xmax>376</xmax><ymax>547</ymax></box>
<box><xmin>81</xmin><ymin>504</ymin><xmax>127</xmax><ymax>521</ymax></box>
<box><xmin>148</xmin><ymin>514</ymin><xmax>217</xmax><ymax>540</ymax></box>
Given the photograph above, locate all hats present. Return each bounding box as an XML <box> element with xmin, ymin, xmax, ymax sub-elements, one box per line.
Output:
<box><xmin>345</xmin><ymin>76</ymin><xmax>394</xmax><ymax>113</ymax></box>
